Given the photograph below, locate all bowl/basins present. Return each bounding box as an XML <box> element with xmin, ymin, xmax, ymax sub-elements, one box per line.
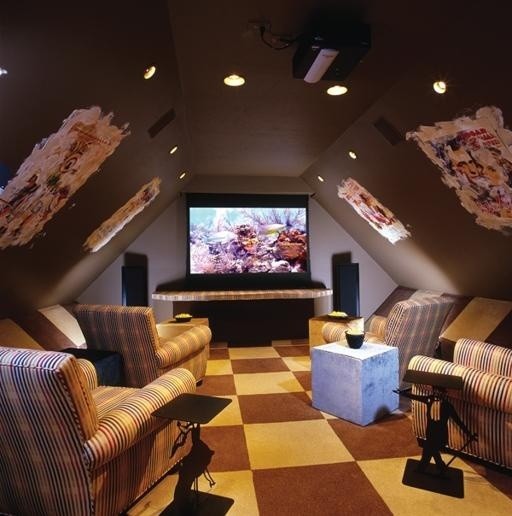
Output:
<box><xmin>344</xmin><ymin>330</ymin><xmax>365</xmax><ymax>350</ymax></box>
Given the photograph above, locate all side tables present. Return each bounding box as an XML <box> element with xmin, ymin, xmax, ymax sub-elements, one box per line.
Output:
<box><xmin>307</xmin><ymin>338</ymin><xmax>400</xmax><ymax>428</ymax></box>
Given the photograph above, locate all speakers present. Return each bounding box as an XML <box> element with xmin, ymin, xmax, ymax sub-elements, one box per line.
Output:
<box><xmin>122</xmin><ymin>265</ymin><xmax>148</xmax><ymax>308</ymax></box>
<box><xmin>333</xmin><ymin>263</ymin><xmax>360</xmax><ymax>318</ymax></box>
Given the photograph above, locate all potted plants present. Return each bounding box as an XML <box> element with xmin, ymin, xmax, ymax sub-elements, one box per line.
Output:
<box><xmin>172</xmin><ymin>313</ymin><xmax>194</xmax><ymax>323</ymax></box>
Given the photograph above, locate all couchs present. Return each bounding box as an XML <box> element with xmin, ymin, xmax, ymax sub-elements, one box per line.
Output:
<box><xmin>1</xmin><ymin>346</ymin><xmax>195</xmax><ymax>515</ymax></box>
<box><xmin>74</xmin><ymin>303</ymin><xmax>212</xmax><ymax>388</ymax></box>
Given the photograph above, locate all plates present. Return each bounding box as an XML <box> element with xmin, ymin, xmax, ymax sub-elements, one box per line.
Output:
<box><xmin>328</xmin><ymin>315</ymin><xmax>348</xmax><ymax>319</ymax></box>
<box><xmin>176</xmin><ymin>317</ymin><xmax>192</xmax><ymax>322</ymax></box>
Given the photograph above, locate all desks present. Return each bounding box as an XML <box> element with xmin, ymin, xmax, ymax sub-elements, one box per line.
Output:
<box><xmin>156</xmin><ymin>318</ymin><xmax>209</xmax><ymax>339</ymax></box>
<box><xmin>147</xmin><ymin>390</ymin><xmax>234</xmax><ymax>516</ymax></box>
<box><xmin>59</xmin><ymin>348</ymin><xmax>126</xmax><ymax>388</ymax></box>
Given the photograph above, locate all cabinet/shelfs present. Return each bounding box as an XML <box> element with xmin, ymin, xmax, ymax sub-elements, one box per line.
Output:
<box><xmin>172</xmin><ymin>296</ymin><xmax>315</xmax><ymax>349</ymax></box>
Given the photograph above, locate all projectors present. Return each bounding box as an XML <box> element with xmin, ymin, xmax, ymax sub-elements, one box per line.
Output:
<box><xmin>293</xmin><ymin>31</ymin><xmax>369</xmax><ymax>81</ymax></box>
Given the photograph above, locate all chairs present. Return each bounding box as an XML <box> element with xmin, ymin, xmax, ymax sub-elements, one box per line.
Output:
<box><xmin>319</xmin><ymin>292</ymin><xmax>512</xmax><ymax>478</ymax></box>
